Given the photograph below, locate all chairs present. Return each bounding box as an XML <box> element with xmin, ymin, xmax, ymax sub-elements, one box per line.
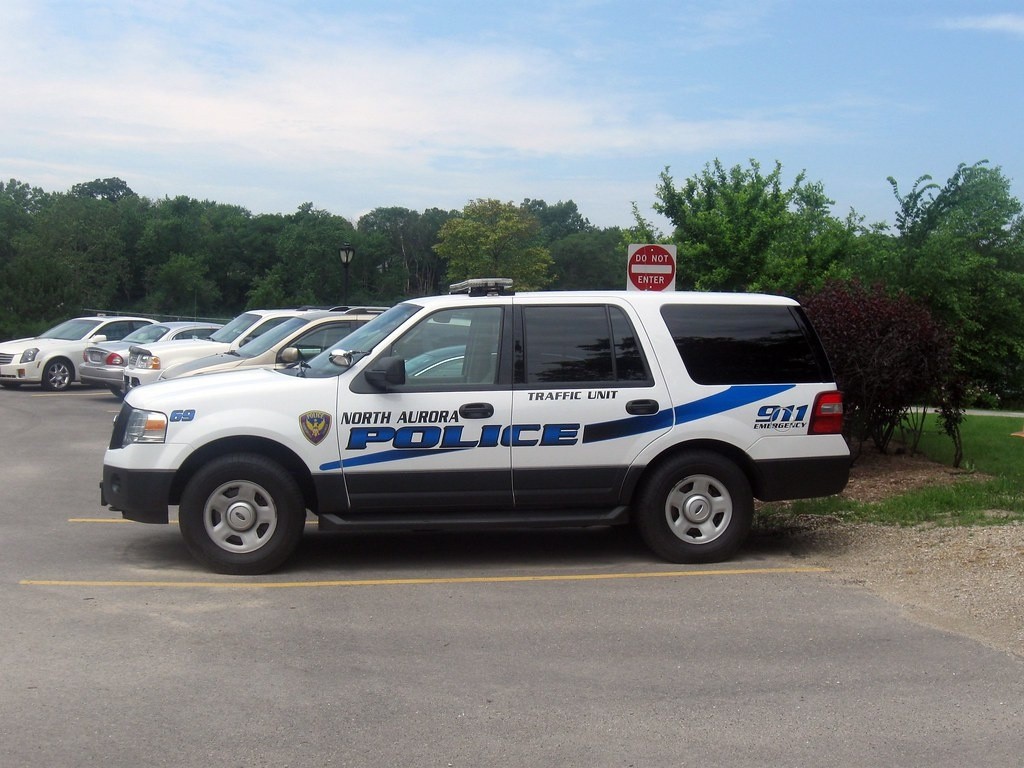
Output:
<box><xmin>138</xmin><ymin>330</ymin><xmax>163</xmax><ymax>340</ymax></box>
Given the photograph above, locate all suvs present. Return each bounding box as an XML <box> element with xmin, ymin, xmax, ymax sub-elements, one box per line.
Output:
<box><xmin>120</xmin><ymin>303</ymin><xmax>347</xmax><ymax>396</ymax></box>
<box><xmin>159</xmin><ymin>306</ymin><xmax>473</xmax><ymax>385</ymax></box>
<box><xmin>99</xmin><ymin>276</ymin><xmax>854</xmax><ymax>575</ymax></box>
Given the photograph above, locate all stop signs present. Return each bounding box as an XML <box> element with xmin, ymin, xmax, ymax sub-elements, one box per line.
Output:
<box><xmin>625</xmin><ymin>243</ymin><xmax>677</xmax><ymax>290</ymax></box>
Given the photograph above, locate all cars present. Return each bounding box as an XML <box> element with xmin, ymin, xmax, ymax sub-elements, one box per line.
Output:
<box><xmin>0</xmin><ymin>315</ymin><xmax>175</xmax><ymax>392</ymax></box>
<box><xmin>78</xmin><ymin>320</ymin><xmax>224</xmax><ymax>400</ymax></box>
<box><xmin>402</xmin><ymin>342</ymin><xmax>645</xmax><ymax>379</ymax></box>
